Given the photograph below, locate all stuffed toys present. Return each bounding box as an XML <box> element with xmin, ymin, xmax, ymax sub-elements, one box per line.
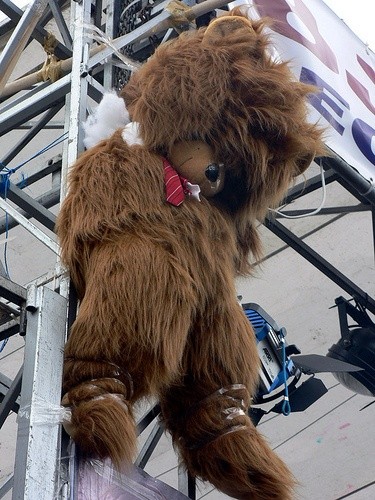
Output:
<box><xmin>53</xmin><ymin>3</ymin><xmax>326</xmax><ymax>500</ymax></box>
